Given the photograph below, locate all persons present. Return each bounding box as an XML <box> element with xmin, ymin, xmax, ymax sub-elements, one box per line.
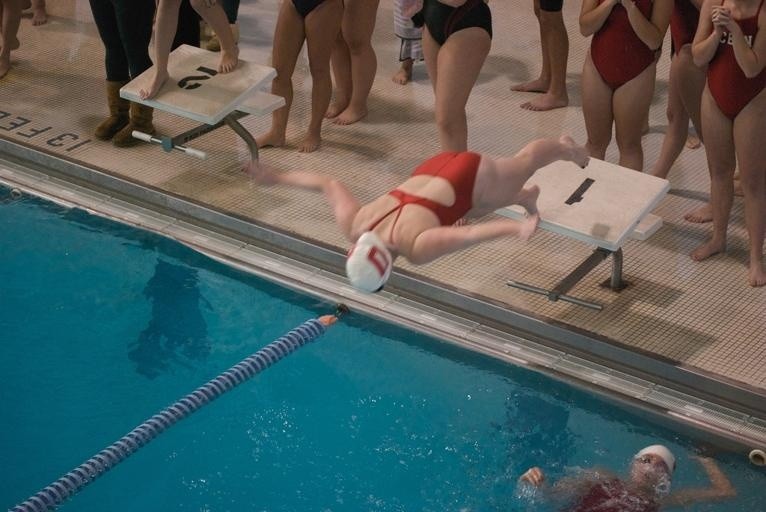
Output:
<box><xmin>1</xmin><ymin>1</ymin><xmax>47</xmax><ymax>79</ymax></box>
<box><xmin>392</xmin><ymin>0</ymin><xmax>492</xmax><ymax>226</ymax></box>
<box><xmin>258</xmin><ymin>137</ymin><xmax>590</xmax><ymax>293</ymax></box>
<box><xmin>578</xmin><ymin>0</ymin><xmax>765</xmax><ymax>287</ymax></box>
<box><xmin>248</xmin><ymin>1</ymin><xmax>379</xmax><ymax>153</ymax></box>
<box><xmin>509</xmin><ymin>0</ymin><xmax>570</xmax><ymax>112</ymax></box>
<box><xmin>89</xmin><ymin>0</ymin><xmax>240</xmax><ymax>148</ymax></box>
<box><xmin>520</xmin><ymin>445</ymin><xmax>737</xmax><ymax>510</ymax></box>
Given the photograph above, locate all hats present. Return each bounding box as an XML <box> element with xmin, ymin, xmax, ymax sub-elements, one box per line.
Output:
<box><xmin>634</xmin><ymin>444</ymin><xmax>676</xmax><ymax>476</ymax></box>
<box><xmin>346</xmin><ymin>230</ymin><xmax>393</xmax><ymax>294</ymax></box>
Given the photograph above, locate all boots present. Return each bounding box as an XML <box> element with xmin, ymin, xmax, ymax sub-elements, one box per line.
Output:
<box><xmin>94</xmin><ymin>75</ymin><xmax>131</xmax><ymax>142</ymax></box>
<box><xmin>111</xmin><ymin>99</ymin><xmax>156</xmax><ymax>148</ymax></box>
